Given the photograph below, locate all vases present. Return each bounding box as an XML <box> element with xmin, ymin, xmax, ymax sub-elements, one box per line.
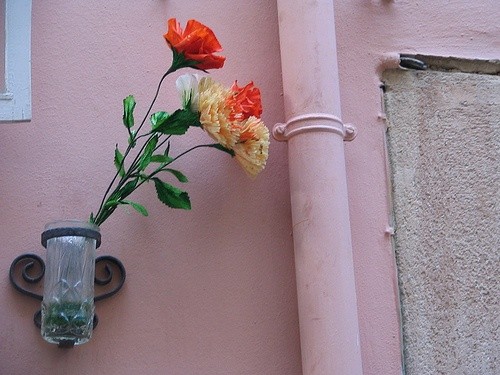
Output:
<box><xmin>40</xmin><ymin>219</ymin><xmax>101</xmax><ymax>345</ymax></box>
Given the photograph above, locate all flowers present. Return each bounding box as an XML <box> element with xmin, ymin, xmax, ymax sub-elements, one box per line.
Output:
<box><xmin>41</xmin><ymin>17</ymin><xmax>272</xmax><ymax>226</ymax></box>
